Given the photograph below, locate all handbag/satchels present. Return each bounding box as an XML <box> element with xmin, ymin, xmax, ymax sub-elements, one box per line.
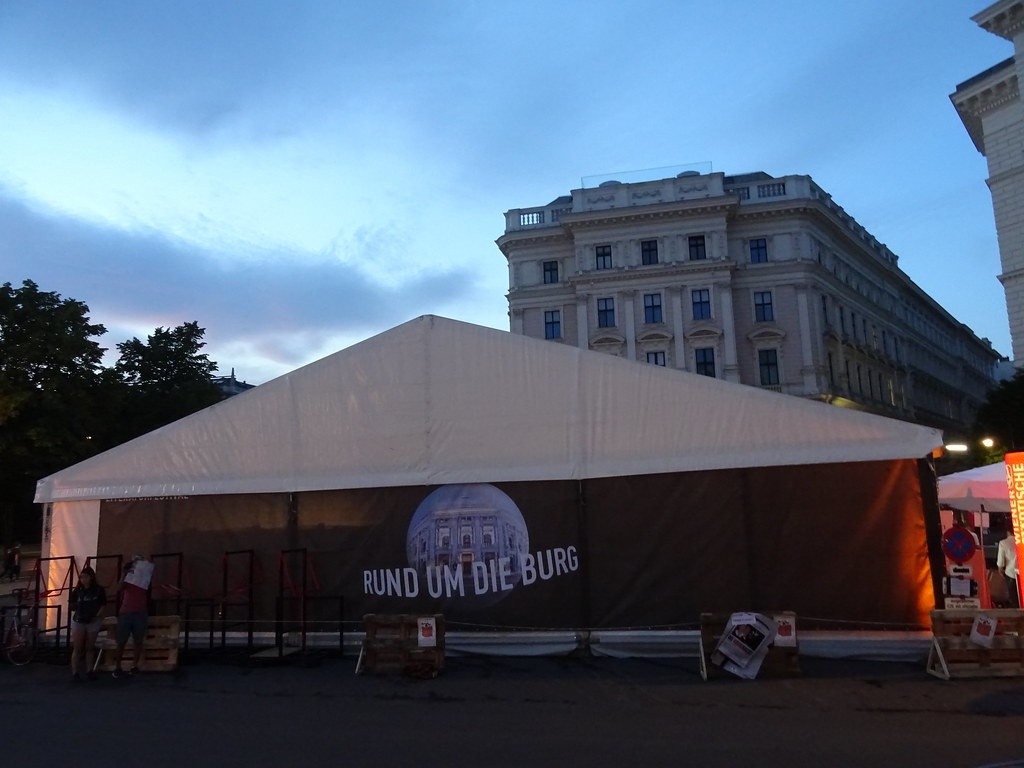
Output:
<box><xmin>73</xmin><ymin>609</ymin><xmax>90</xmax><ymax>623</ymax></box>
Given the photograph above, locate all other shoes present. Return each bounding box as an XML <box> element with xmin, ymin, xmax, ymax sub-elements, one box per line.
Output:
<box><xmin>72</xmin><ymin>672</ymin><xmax>80</xmax><ymax>681</ymax></box>
<box><xmin>128</xmin><ymin>666</ymin><xmax>138</xmax><ymax>678</ymax></box>
<box><xmin>88</xmin><ymin>671</ymin><xmax>97</xmax><ymax>680</ymax></box>
<box><xmin>111</xmin><ymin>669</ymin><xmax>120</xmax><ymax>680</ymax></box>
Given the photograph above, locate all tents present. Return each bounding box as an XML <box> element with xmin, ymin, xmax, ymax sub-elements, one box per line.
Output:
<box><xmin>936</xmin><ymin>460</ymin><xmax>1011</xmax><ymax>609</ymax></box>
<box><xmin>32</xmin><ymin>312</ymin><xmax>947</xmax><ymax>667</ymax></box>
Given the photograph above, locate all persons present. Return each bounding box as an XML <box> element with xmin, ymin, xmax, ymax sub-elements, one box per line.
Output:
<box><xmin>71</xmin><ymin>567</ymin><xmax>108</xmax><ymax>680</ymax></box>
<box><xmin>111</xmin><ymin>551</ymin><xmax>149</xmax><ymax>680</ymax></box>
<box><xmin>994</xmin><ymin>534</ymin><xmax>1020</xmax><ymax>609</ymax></box>
<box><xmin>0</xmin><ymin>543</ymin><xmax>24</xmax><ymax>582</ymax></box>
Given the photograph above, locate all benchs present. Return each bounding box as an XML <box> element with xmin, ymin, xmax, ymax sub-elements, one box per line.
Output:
<box><xmin>699</xmin><ymin>613</ymin><xmax>803</xmax><ymax>682</ymax></box>
<box><xmin>93</xmin><ymin>615</ymin><xmax>180</xmax><ymax>672</ymax></box>
<box><xmin>354</xmin><ymin>614</ymin><xmax>446</xmax><ymax>679</ymax></box>
<box><xmin>926</xmin><ymin>608</ymin><xmax>1024</xmax><ymax>679</ymax></box>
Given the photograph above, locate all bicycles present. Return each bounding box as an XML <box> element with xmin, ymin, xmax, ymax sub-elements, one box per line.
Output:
<box><xmin>0</xmin><ymin>587</ymin><xmax>39</xmax><ymax>667</ymax></box>
